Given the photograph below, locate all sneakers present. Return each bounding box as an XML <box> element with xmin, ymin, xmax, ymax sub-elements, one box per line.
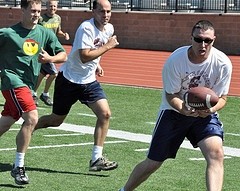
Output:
<box><xmin>89</xmin><ymin>157</ymin><xmax>118</xmax><ymax>171</ymax></box>
<box><xmin>32</xmin><ymin>93</ymin><xmax>39</xmax><ymax>106</ymax></box>
<box><xmin>11</xmin><ymin>163</ymin><xmax>29</xmax><ymax>185</ymax></box>
<box><xmin>40</xmin><ymin>93</ymin><xmax>53</xmax><ymax>106</ymax></box>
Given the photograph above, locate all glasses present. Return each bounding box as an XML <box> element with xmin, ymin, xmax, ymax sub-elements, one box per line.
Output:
<box><xmin>192</xmin><ymin>35</ymin><xmax>214</xmax><ymax>43</ymax></box>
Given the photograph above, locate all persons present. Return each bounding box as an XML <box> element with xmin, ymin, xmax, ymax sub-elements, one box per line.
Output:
<box><xmin>28</xmin><ymin>0</ymin><xmax>119</xmax><ymax>172</ymax></box>
<box><xmin>118</xmin><ymin>20</ymin><xmax>232</xmax><ymax>191</ymax></box>
<box><xmin>0</xmin><ymin>0</ymin><xmax>68</xmax><ymax>185</ymax></box>
<box><xmin>30</xmin><ymin>0</ymin><xmax>70</xmax><ymax>106</ymax></box>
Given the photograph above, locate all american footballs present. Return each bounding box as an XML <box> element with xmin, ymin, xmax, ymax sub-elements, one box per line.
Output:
<box><xmin>183</xmin><ymin>86</ymin><xmax>218</xmax><ymax>110</ymax></box>
